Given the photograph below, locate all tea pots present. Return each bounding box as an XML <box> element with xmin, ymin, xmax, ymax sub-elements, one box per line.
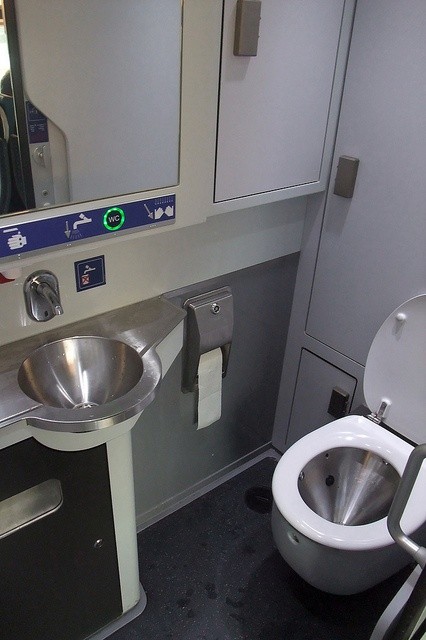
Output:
<box><xmin>0</xmin><ymin>2</ymin><xmax>184</xmax><ymax>221</ymax></box>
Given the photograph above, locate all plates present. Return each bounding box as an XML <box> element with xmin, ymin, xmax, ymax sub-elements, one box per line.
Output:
<box><xmin>213</xmin><ymin>1</ymin><xmax>347</xmax><ymax>203</ymax></box>
<box><xmin>285</xmin><ymin>349</ymin><xmax>358</xmax><ymax>449</ymax></box>
<box><xmin>304</xmin><ymin>0</ymin><xmax>426</xmax><ymax>370</ymax></box>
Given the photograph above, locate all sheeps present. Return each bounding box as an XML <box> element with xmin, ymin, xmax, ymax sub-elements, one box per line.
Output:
<box><xmin>22</xmin><ymin>269</ymin><xmax>64</xmax><ymax>324</ymax></box>
